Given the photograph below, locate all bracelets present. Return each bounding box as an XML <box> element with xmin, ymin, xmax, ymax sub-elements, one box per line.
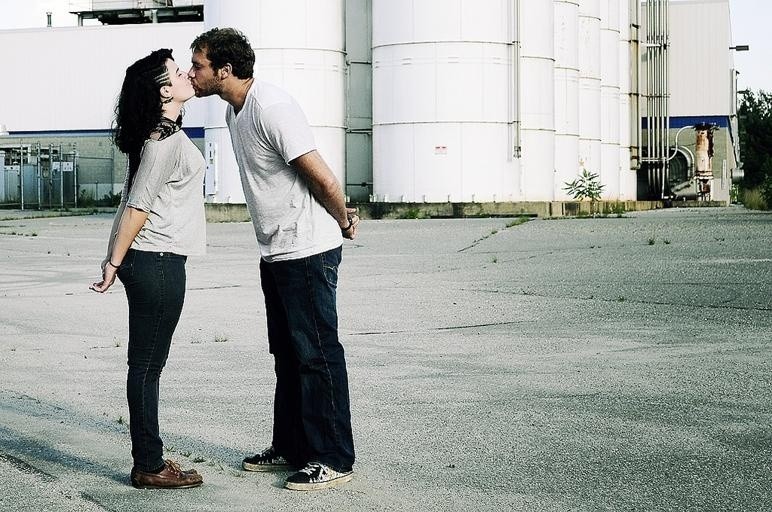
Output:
<box><xmin>109</xmin><ymin>257</ymin><xmax>121</xmax><ymax>268</ymax></box>
<box><xmin>340</xmin><ymin>212</ymin><xmax>353</xmax><ymax>231</ymax></box>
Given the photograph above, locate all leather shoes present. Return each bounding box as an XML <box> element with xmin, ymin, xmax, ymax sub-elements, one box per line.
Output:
<box><xmin>132</xmin><ymin>458</ymin><xmax>203</xmax><ymax>489</ymax></box>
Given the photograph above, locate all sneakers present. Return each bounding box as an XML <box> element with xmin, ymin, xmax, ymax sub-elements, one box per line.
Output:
<box><xmin>284</xmin><ymin>460</ymin><xmax>354</xmax><ymax>490</ymax></box>
<box><xmin>242</xmin><ymin>446</ymin><xmax>293</xmax><ymax>471</ymax></box>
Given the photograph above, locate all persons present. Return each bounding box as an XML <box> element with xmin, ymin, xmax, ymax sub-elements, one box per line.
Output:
<box><xmin>86</xmin><ymin>46</ymin><xmax>205</xmax><ymax>491</ymax></box>
<box><xmin>187</xmin><ymin>25</ymin><xmax>363</xmax><ymax>493</ymax></box>
